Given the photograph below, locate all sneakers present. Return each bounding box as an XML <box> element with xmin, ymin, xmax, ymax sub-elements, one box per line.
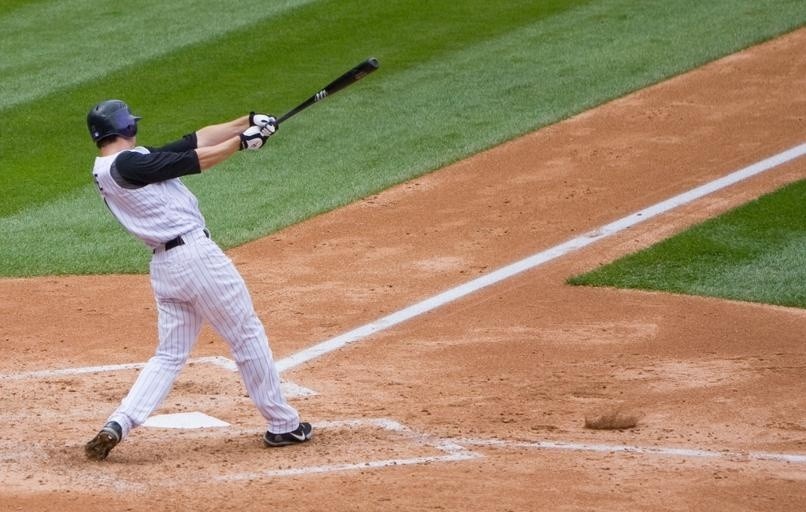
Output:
<box><xmin>86</xmin><ymin>422</ymin><xmax>123</xmax><ymax>463</ymax></box>
<box><xmin>264</xmin><ymin>422</ymin><xmax>312</xmax><ymax>446</ymax></box>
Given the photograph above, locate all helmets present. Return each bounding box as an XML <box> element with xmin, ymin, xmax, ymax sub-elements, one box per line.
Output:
<box><xmin>87</xmin><ymin>100</ymin><xmax>143</xmax><ymax>140</ymax></box>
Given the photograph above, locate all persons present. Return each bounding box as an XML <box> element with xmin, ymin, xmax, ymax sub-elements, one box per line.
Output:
<box><xmin>84</xmin><ymin>97</ymin><xmax>311</xmax><ymax>461</ymax></box>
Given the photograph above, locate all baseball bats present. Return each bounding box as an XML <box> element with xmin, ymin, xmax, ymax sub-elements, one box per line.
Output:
<box><xmin>273</xmin><ymin>58</ymin><xmax>380</xmax><ymax>127</ymax></box>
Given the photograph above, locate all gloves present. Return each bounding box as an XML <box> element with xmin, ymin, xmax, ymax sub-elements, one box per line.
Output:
<box><xmin>239</xmin><ymin>111</ymin><xmax>278</xmax><ymax>151</ymax></box>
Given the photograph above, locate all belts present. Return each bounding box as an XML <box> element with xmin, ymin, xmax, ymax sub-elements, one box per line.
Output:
<box><xmin>166</xmin><ymin>228</ymin><xmax>209</xmax><ymax>250</ymax></box>
